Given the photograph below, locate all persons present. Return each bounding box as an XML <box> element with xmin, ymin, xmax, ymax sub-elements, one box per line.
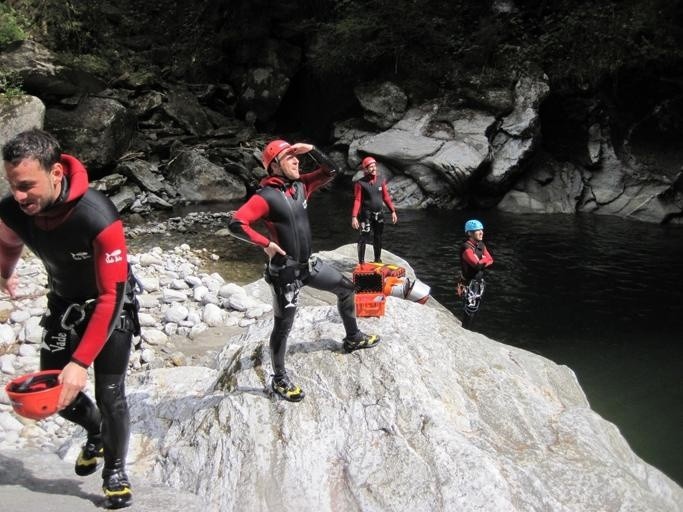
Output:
<box><xmin>351</xmin><ymin>156</ymin><xmax>397</xmax><ymax>264</ymax></box>
<box><xmin>227</xmin><ymin>139</ymin><xmax>381</xmax><ymax>402</ymax></box>
<box><xmin>0</xmin><ymin>129</ymin><xmax>144</xmax><ymax>510</ymax></box>
<box><xmin>456</xmin><ymin>219</ymin><xmax>493</xmax><ymax>329</ymax></box>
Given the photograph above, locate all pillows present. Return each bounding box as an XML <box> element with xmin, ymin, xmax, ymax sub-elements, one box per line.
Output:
<box><xmin>464</xmin><ymin>219</ymin><xmax>484</xmax><ymax>234</ymax></box>
<box><xmin>260</xmin><ymin>137</ymin><xmax>291</xmax><ymax>170</ymax></box>
<box><xmin>362</xmin><ymin>156</ymin><xmax>376</xmax><ymax>168</ymax></box>
<box><xmin>5</xmin><ymin>369</ymin><xmax>63</xmax><ymax>422</ymax></box>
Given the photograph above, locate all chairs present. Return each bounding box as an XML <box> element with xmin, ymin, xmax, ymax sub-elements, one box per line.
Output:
<box><xmin>100</xmin><ymin>457</ymin><xmax>134</xmax><ymax>509</ymax></box>
<box><xmin>74</xmin><ymin>428</ymin><xmax>104</xmax><ymax>476</ymax></box>
<box><xmin>270</xmin><ymin>372</ymin><xmax>306</xmax><ymax>402</ymax></box>
<box><xmin>341</xmin><ymin>330</ymin><xmax>381</xmax><ymax>353</ymax></box>
